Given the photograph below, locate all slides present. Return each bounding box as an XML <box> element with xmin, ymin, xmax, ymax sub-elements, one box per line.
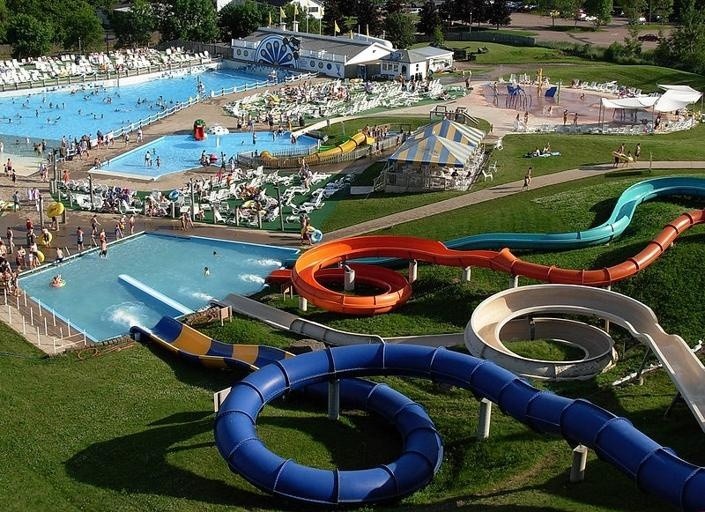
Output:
<box><xmin>194</xmin><ymin>127</ymin><xmax>206</xmax><ymax>141</ymax></box>
<box><xmin>263</xmin><ymin>209</ymin><xmax>705</xmax><ymax>316</ymax></box>
<box><xmin>209</xmin><ymin>282</ymin><xmax>705</xmax><ymax>436</ymax></box>
<box><xmin>130</xmin><ymin>316</ymin><xmax>705</xmax><ymax>512</ymax></box>
<box><xmin>260</xmin><ymin>132</ymin><xmax>375</xmax><ymax>166</ymax></box>
<box><xmin>277</xmin><ymin>176</ymin><xmax>704</xmax><ymax>270</ymax></box>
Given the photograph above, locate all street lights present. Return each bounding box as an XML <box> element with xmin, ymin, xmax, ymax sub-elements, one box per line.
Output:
<box><xmin>274</xmin><ymin>182</ymin><xmax>284</xmax><ymax>232</ymax></box>
<box><xmin>104</xmin><ymin>29</ymin><xmax>114</xmax><ymax>55</ymax></box>
<box><xmin>469</xmin><ymin>10</ymin><xmax>473</xmax><ymax>32</ymax></box>
<box><xmin>184</xmin><ymin>182</ymin><xmax>195</xmax><ymax>222</ymax></box>
<box><xmin>52</xmin><ymin>148</ymin><xmax>59</xmax><ymax>180</ymax></box>
<box><xmin>86</xmin><ymin>173</ymin><xmax>93</xmax><ymax>212</ymax></box>
<box><xmin>448</xmin><ymin>13</ymin><xmax>451</xmax><ymax>33</ymax></box>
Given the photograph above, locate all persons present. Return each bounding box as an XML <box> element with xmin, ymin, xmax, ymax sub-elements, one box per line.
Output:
<box><xmin>523</xmin><ymin>167</ymin><xmax>533</xmax><ymax>186</ymax></box>
<box><xmin>573</xmin><ymin>112</ymin><xmax>578</xmax><ymax>126</ymax></box>
<box><xmin>634</xmin><ymin>143</ymin><xmax>641</xmax><ymax>162</ymax></box>
<box><xmin>622</xmin><ymin>151</ymin><xmax>632</xmax><ymax>168</ymax></box>
<box><xmin>612</xmin><ymin>143</ymin><xmax>626</xmax><ymax>168</ymax></box>
<box><xmin>1</xmin><ymin>47</ymin><xmax>439</xmax><ymax>296</ymax></box>
<box><xmin>564</xmin><ymin>110</ymin><xmax>569</xmax><ymax>125</ymax></box>
<box><xmin>494</xmin><ymin>82</ymin><xmax>498</xmax><ymax>94</ymax></box>
<box><xmin>545</xmin><ymin>141</ymin><xmax>552</xmax><ymax>154</ymax></box>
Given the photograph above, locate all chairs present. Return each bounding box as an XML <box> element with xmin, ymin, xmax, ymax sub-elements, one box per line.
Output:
<box><xmin>57</xmin><ymin>169</ymin><xmax>350</xmax><ymax>230</ymax></box>
<box><xmin>221</xmin><ymin>76</ymin><xmax>456</xmax><ymax>125</ymax></box>
<box><xmin>495</xmin><ymin>70</ymin><xmax>700</xmax><ymax>137</ymax></box>
<box><xmin>0</xmin><ymin>46</ymin><xmax>214</xmax><ymax>87</ymax></box>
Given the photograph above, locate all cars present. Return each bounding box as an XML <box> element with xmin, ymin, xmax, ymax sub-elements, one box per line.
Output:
<box><xmin>550</xmin><ymin>9</ymin><xmax>599</xmax><ymax>21</ymax></box>
<box><xmin>373</xmin><ymin>3</ymin><xmax>423</xmax><ymax>17</ymax></box>
<box><xmin>638</xmin><ymin>33</ymin><xmax>659</xmax><ymax>42</ymax></box>
<box><xmin>505</xmin><ymin>0</ymin><xmax>538</xmax><ymax>13</ymax></box>
<box><xmin>609</xmin><ymin>10</ymin><xmax>663</xmax><ymax>25</ymax></box>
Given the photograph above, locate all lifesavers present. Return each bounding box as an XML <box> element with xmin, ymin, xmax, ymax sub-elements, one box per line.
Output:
<box><xmin>612</xmin><ymin>150</ymin><xmax>633</xmax><ymax>162</ymax></box>
<box><xmin>47</xmin><ymin>202</ymin><xmax>64</xmax><ymax>217</ymax></box>
<box><xmin>37</xmin><ymin>250</ymin><xmax>45</xmax><ymax>262</ymax></box>
<box><xmin>50</xmin><ymin>280</ymin><xmax>66</xmax><ymax>288</ymax></box>
<box><xmin>207</xmin><ymin>124</ymin><xmax>229</xmax><ymax>134</ymax></box>
<box><xmin>38</xmin><ymin>232</ymin><xmax>52</xmax><ymax>245</ymax></box>
